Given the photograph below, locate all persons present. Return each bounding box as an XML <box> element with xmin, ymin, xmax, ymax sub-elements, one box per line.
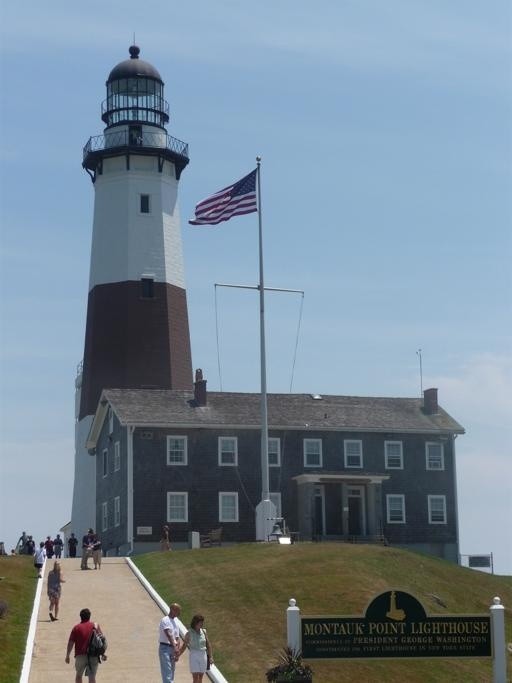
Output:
<box><xmin>65</xmin><ymin>609</ymin><xmax>107</xmax><ymax>683</ymax></box>
<box><xmin>175</xmin><ymin>615</ymin><xmax>214</xmax><ymax>683</ymax></box>
<box><xmin>0</xmin><ymin>529</ymin><xmax>102</xmax><ymax>578</ymax></box>
<box><xmin>47</xmin><ymin>561</ymin><xmax>66</xmax><ymax>621</ymax></box>
<box><xmin>159</xmin><ymin>603</ymin><xmax>181</xmax><ymax>683</ymax></box>
<box><xmin>160</xmin><ymin>525</ymin><xmax>171</xmax><ymax>551</ymax></box>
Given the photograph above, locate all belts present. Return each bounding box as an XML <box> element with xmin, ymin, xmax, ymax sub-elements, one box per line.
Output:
<box><xmin>160</xmin><ymin>641</ymin><xmax>173</xmax><ymax>647</ymax></box>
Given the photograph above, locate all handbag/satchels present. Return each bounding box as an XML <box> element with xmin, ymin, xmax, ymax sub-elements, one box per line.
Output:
<box><xmin>206</xmin><ymin>654</ymin><xmax>211</xmax><ymax>671</ymax></box>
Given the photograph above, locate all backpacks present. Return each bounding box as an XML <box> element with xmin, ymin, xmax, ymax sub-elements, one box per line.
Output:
<box><xmin>86</xmin><ymin>622</ymin><xmax>109</xmax><ymax>659</ymax></box>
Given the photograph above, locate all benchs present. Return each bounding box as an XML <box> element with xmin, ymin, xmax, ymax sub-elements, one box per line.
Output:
<box><xmin>201</xmin><ymin>525</ymin><xmax>224</xmax><ymax>548</ymax></box>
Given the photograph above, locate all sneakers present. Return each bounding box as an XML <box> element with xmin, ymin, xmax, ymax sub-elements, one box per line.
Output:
<box><xmin>48</xmin><ymin>612</ymin><xmax>60</xmax><ymax>622</ymax></box>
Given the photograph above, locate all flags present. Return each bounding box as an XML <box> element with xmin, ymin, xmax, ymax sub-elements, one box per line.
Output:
<box><xmin>189</xmin><ymin>168</ymin><xmax>258</xmax><ymax>225</ymax></box>
